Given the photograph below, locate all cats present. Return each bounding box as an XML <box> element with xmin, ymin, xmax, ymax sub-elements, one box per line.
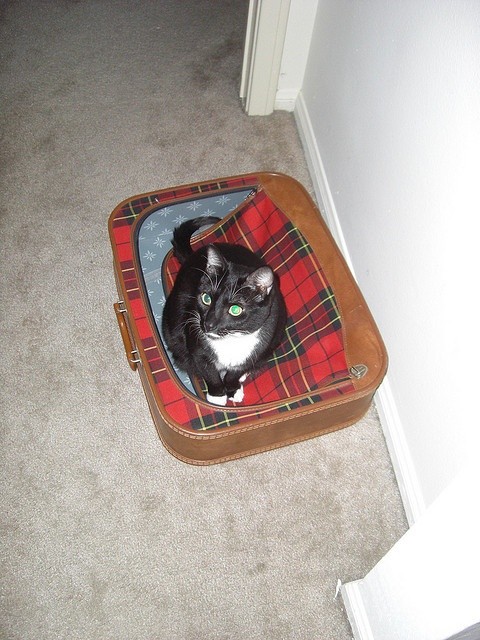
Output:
<box><xmin>160</xmin><ymin>215</ymin><xmax>288</xmax><ymax>407</ymax></box>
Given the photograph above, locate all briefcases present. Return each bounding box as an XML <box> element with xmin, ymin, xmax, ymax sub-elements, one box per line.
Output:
<box><xmin>108</xmin><ymin>171</ymin><xmax>388</xmax><ymax>466</ymax></box>
